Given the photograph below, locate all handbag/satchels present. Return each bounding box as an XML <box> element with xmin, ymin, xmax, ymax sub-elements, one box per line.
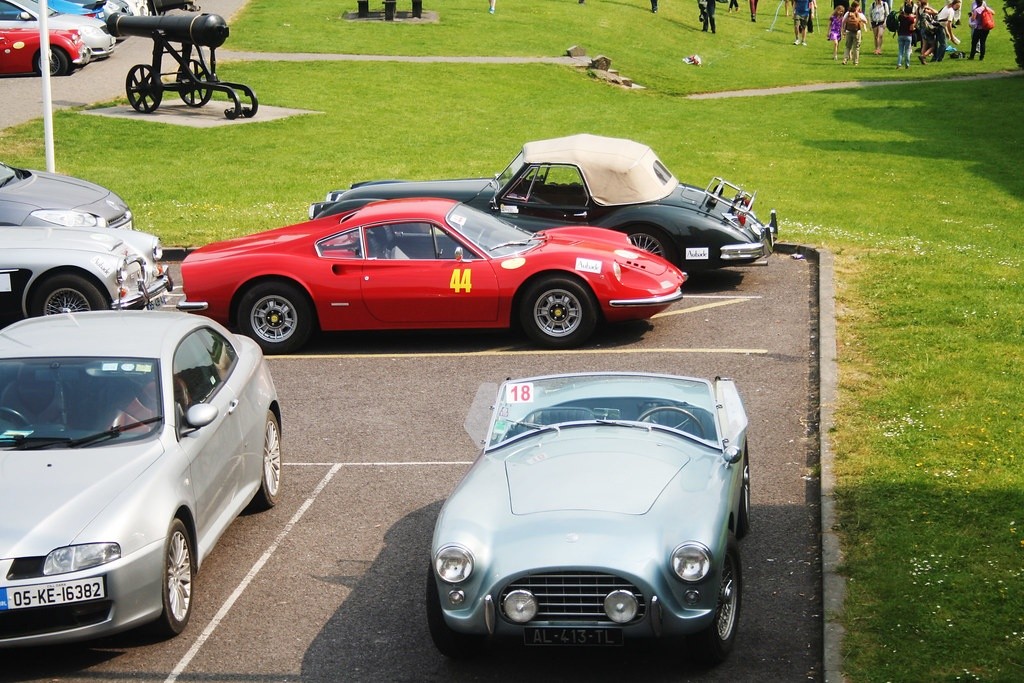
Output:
<box><xmin>807</xmin><ymin>20</ymin><xmax>813</xmax><ymax>33</ymax></box>
<box><xmin>911</xmin><ymin>32</ymin><xmax>917</xmax><ymax>47</ymax></box>
<box><xmin>886</xmin><ymin>11</ymin><xmax>900</xmax><ymax>32</ymax></box>
<box><xmin>982</xmin><ymin>9</ymin><xmax>994</xmax><ymax>31</ymax></box>
<box><xmin>915</xmin><ymin>28</ymin><xmax>922</xmax><ymax>41</ymax></box>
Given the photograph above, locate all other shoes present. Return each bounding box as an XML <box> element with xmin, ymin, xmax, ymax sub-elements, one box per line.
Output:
<box><xmin>736</xmin><ymin>8</ymin><xmax>739</xmax><ymax>11</ymax></box>
<box><xmin>752</xmin><ymin>14</ymin><xmax>756</xmax><ymax>22</ymax></box>
<box><xmin>652</xmin><ymin>7</ymin><xmax>657</xmax><ymax>13</ymax></box>
<box><xmin>489</xmin><ymin>7</ymin><xmax>495</xmax><ymax>14</ymax></box>
<box><xmin>833</xmin><ymin>49</ymin><xmax>984</xmax><ymax>70</ymax></box>
<box><xmin>802</xmin><ymin>42</ymin><xmax>807</xmax><ymax>46</ymax></box>
<box><xmin>794</xmin><ymin>40</ymin><xmax>800</xmax><ymax>45</ymax></box>
<box><xmin>729</xmin><ymin>8</ymin><xmax>732</xmax><ymax>13</ymax></box>
<box><xmin>702</xmin><ymin>29</ymin><xmax>707</xmax><ymax>31</ymax></box>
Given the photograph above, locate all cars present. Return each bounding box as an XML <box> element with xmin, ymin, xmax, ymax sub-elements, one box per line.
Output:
<box><xmin>0</xmin><ymin>228</ymin><xmax>172</xmax><ymax>326</ymax></box>
<box><xmin>182</xmin><ymin>196</ymin><xmax>690</xmax><ymax>366</ymax></box>
<box><xmin>426</xmin><ymin>373</ymin><xmax>753</xmax><ymax>666</ymax></box>
<box><xmin>0</xmin><ymin>306</ymin><xmax>284</xmax><ymax>659</ymax></box>
<box><xmin>0</xmin><ymin>0</ymin><xmax>135</xmax><ymax>78</ymax></box>
<box><xmin>0</xmin><ymin>162</ymin><xmax>136</xmax><ymax>231</ymax></box>
<box><xmin>315</xmin><ymin>133</ymin><xmax>778</xmax><ymax>289</ymax></box>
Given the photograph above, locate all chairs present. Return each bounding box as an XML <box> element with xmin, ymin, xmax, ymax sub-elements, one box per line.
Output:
<box><xmin>649</xmin><ymin>406</ymin><xmax>718</xmax><ymax>442</ymax></box>
<box><xmin>356</xmin><ymin>224</ymin><xmax>416</xmax><ymax>260</ymax></box>
<box><xmin>516</xmin><ymin>406</ymin><xmax>594</xmax><ymax>437</ymax></box>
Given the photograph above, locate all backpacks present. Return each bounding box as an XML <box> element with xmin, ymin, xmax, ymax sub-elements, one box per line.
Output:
<box><xmin>846</xmin><ymin>12</ymin><xmax>859</xmax><ymax>31</ymax></box>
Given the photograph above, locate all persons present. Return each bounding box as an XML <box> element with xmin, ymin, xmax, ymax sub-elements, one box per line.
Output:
<box><xmin>828</xmin><ymin>0</ymin><xmax>867</xmax><ymax>65</ymax></box>
<box><xmin>749</xmin><ymin>0</ymin><xmax>758</xmax><ymax>22</ymax></box>
<box><xmin>785</xmin><ymin>0</ymin><xmax>817</xmax><ymax>46</ymax></box>
<box><xmin>728</xmin><ymin>0</ymin><xmax>740</xmax><ymax>13</ymax></box>
<box><xmin>967</xmin><ymin>0</ymin><xmax>995</xmax><ymax>61</ymax></box>
<box><xmin>702</xmin><ymin>0</ymin><xmax>716</xmax><ymax>34</ymax></box>
<box><xmin>488</xmin><ymin>0</ymin><xmax>496</xmax><ymax>14</ymax></box>
<box><xmin>868</xmin><ymin>0</ymin><xmax>892</xmax><ymax>55</ymax></box>
<box><xmin>651</xmin><ymin>0</ymin><xmax>658</xmax><ymax>13</ymax></box>
<box><xmin>896</xmin><ymin>0</ymin><xmax>962</xmax><ymax>69</ymax></box>
